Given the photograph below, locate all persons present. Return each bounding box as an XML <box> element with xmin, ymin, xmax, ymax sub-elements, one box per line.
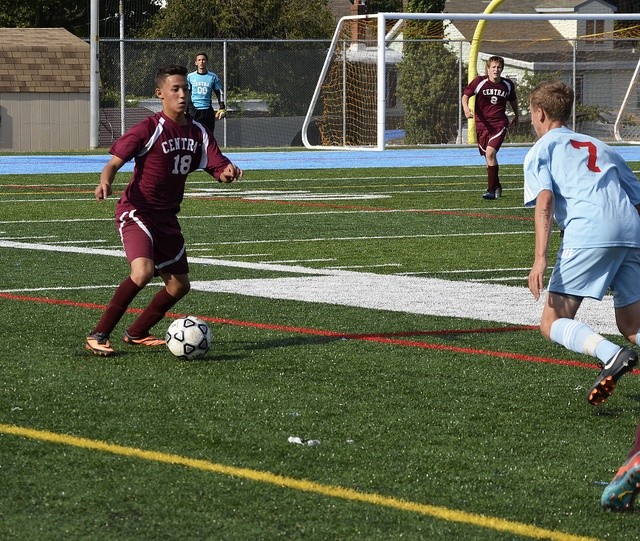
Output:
<box><xmin>186</xmin><ymin>53</ymin><xmax>228</xmax><ymax>138</ymax></box>
<box><xmin>523</xmin><ymin>82</ymin><xmax>640</xmax><ymax>402</ymax></box>
<box><xmin>462</xmin><ymin>56</ymin><xmax>519</xmax><ymax>199</ymax></box>
<box><xmin>600</xmin><ymin>422</ymin><xmax>640</xmax><ymax>510</ymax></box>
<box><xmin>84</xmin><ymin>65</ymin><xmax>242</xmax><ymax>356</ymax></box>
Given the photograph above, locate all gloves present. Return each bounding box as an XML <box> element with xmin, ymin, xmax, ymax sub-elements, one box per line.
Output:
<box><xmin>214</xmin><ymin>104</ymin><xmax>229</xmax><ymax>124</ymax></box>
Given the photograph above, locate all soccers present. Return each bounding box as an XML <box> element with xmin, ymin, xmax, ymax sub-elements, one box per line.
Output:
<box><xmin>165</xmin><ymin>315</ymin><xmax>211</xmax><ymax>360</ymax></box>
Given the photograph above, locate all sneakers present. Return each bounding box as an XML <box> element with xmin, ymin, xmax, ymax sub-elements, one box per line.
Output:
<box><xmin>86</xmin><ymin>331</ymin><xmax>115</xmax><ymax>357</ymax></box>
<box><xmin>586</xmin><ymin>345</ymin><xmax>638</xmax><ymax>406</ymax></box>
<box><xmin>120</xmin><ymin>330</ymin><xmax>166</xmax><ymax>346</ymax></box>
<box><xmin>601</xmin><ymin>451</ymin><xmax>640</xmax><ymax>512</ymax></box>
<box><xmin>495</xmin><ymin>183</ymin><xmax>502</xmax><ymax>199</ymax></box>
<box><xmin>483</xmin><ymin>190</ymin><xmax>495</xmax><ymax>200</ymax></box>
